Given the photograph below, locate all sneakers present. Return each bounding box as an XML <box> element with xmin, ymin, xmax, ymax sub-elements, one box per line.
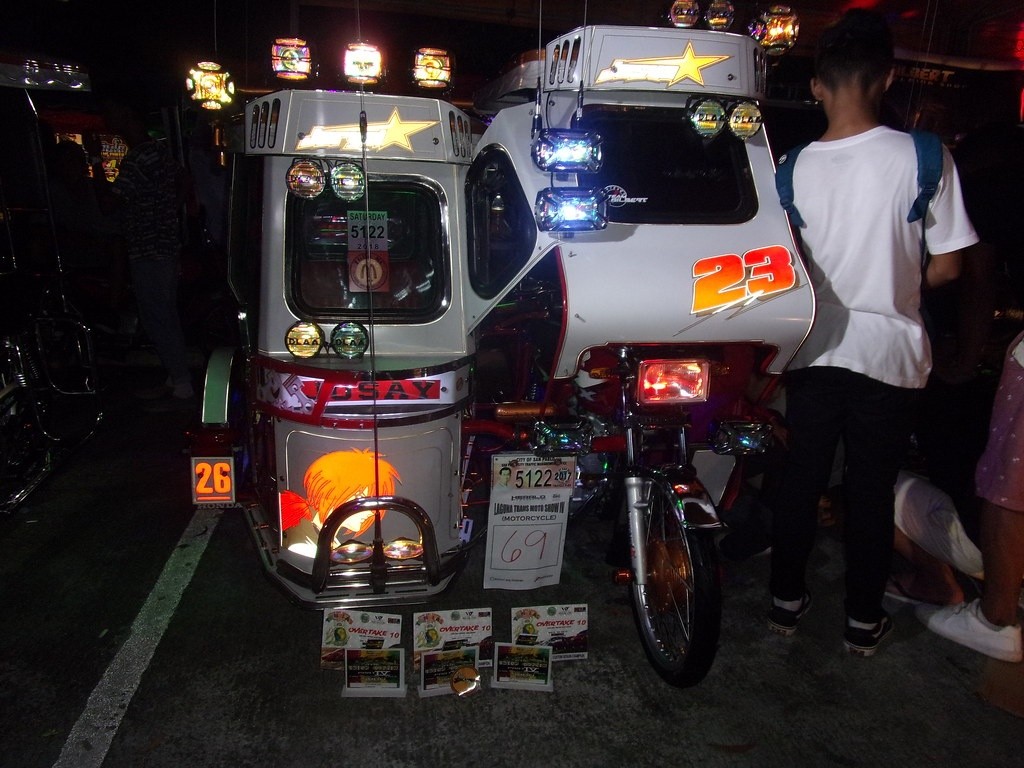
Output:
<box><xmin>141</xmin><ymin>380</ymin><xmax>193</xmax><ymax>401</ymax></box>
<box><xmin>844</xmin><ymin>610</ymin><xmax>895</xmax><ymax>658</ymax></box>
<box><xmin>769</xmin><ymin>591</ymin><xmax>814</xmax><ymax>636</ymax></box>
<box><xmin>915</xmin><ymin>598</ymin><xmax>1022</xmax><ymax>662</ymax></box>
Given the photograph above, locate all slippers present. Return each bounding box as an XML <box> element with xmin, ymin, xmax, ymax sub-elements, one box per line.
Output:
<box><xmin>885</xmin><ymin>576</ymin><xmax>964</xmax><ymax>608</ymax></box>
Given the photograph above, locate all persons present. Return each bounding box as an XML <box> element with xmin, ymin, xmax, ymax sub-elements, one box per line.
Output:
<box><xmin>912</xmin><ymin>328</ymin><xmax>1024</xmax><ymax>662</ymax></box>
<box><xmin>766</xmin><ymin>8</ymin><xmax>980</xmax><ymax>658</ymax></box>
<box><xmin>82</xmin><ymin>102</ymin><xmax>196</xmax><ymax>400</ymax></box>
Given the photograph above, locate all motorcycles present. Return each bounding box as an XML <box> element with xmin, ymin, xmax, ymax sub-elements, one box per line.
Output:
<box><xmin>182</xmin><ymin>1</ymin><xmax>817</xmax><ymax>686</ymax></box>
